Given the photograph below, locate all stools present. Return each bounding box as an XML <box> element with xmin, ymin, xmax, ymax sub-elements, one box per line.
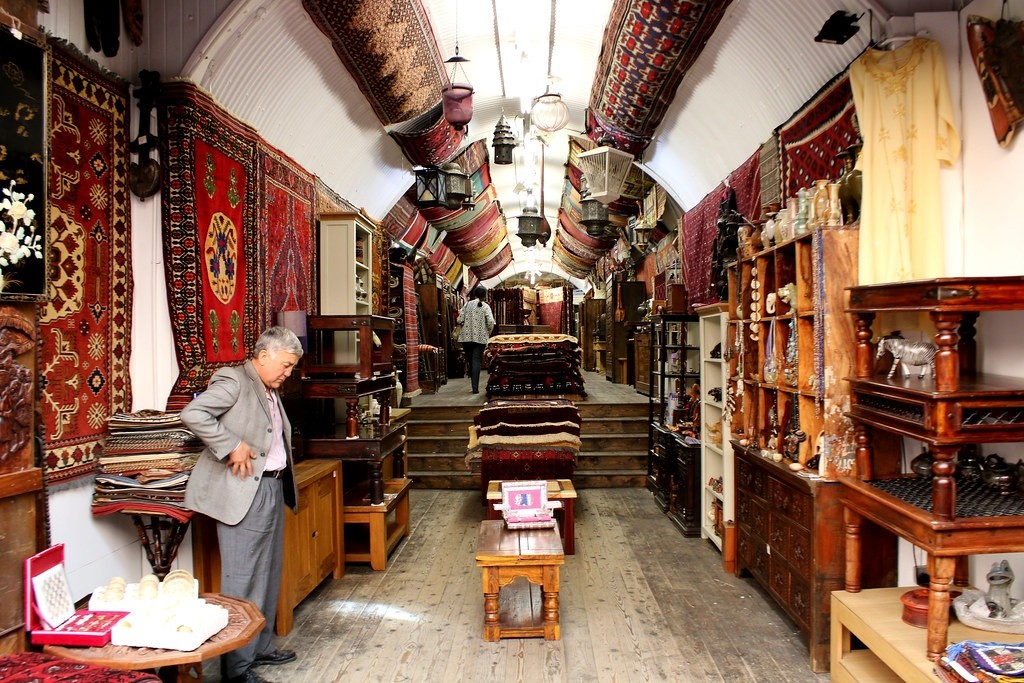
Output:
<box><xmin>416</xmin><ymin>344</ymin><xmax>439</xmax><ymax>396</ymax></box>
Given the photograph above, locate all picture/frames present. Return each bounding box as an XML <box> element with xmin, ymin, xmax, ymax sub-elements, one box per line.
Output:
<box><xmin>0</xmin><ymin>10</ymin><xmax>51</xmax><ymax>304</ymax></box>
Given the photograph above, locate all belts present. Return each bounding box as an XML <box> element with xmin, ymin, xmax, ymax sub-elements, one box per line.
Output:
<box><xmin>262</xmin><ymin>470</ymin><xmax>283</xmax><ymax>479</ymax></box>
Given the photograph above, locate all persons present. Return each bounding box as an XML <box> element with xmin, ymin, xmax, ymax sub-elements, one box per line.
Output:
<box><xmin>456</xmin><ymin>287</ymin><xmax>494</xmax><ymax>394</ymax></box>
<box><xmin>181</xmin><ymin>326</ymin><xmax>303</xmax><ymax>683</ymax></box>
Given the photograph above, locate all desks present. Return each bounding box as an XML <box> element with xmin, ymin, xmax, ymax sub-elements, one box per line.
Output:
<box><xmin>474</xmin><ymin>479</ymin><xmax>578</xmax><ymax>642</ymax></box>
<box><xmin>42</xmin><ymin>593</ymin><xmax>267</xmax><ymax>683</ymax></box>
<box><xmin>300</xmin><ymin>314</ymin><xmax>413</xmax><ymax>571</ymax></box>
<box><xmin>118</xmin><ymin>510</ymin><xmax>191</xmax><ymax>582</ymax></box>
<box><xmin>498</xmin><ymin>324</ymin><xmax>552</xmax><ymax>334</ymax></box>
<box><xmin>831</xmin><ymin>275</ymin><xmax>1024</xmax><ymax>683</ymax></box>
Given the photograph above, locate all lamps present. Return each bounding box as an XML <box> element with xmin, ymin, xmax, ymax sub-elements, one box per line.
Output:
<box><xmin>815</xmin><ymin>10</ymin><xmax>860</xmax><ymax>46</ymax></box>
<box><xmin>531</xmin><ymin>94</ymin><xmax>570</xmax><ymax>132</ymax></box>
<box><xmin>413</xmin><ymin>163</ymin><xmax>450</xmax><ymax>210</ymax></box>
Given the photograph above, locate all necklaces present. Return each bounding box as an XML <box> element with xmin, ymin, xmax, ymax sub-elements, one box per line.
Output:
<box><xmin>811</xmin><ymin>234</ymin><xmax>822</xmax><ymax>419</ymax></box>
<box><xmin>792</xmin><ymin>393</ymin><xmax>800</xmax><ymax>430</ymax></box>
<box><xmin>783</xmin><ymin>314</ymin><xmax>797</xmax><ymax>386</ymax></box>
<box><xmin>735</xmin><ymin>321</ymin><xmax>744</xmax><ymax>379</ymax></box>
<box><xmin>771</xmin><ymin>388</ymin><xmax>779</xmax><ymax>437</ymax></box>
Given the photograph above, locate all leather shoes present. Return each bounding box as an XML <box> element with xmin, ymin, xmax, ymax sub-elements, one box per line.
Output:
<box><xmin>221</xmin><ymin>667</ymin><xmax>271</xmax><ymax>683</ymax></box>
<box><xmin>251</xmin><ymin>649</ymin><xmax>297</xmax><ymax>667</ymax></box>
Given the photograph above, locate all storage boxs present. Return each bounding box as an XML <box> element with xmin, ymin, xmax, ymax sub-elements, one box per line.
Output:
<box><xmin>721</xmin><ymin>519</ymin><xmax>734</xmax><ymax>574</ymax></box>
<box><xmin>23</xmin><ymin>543</ymin><xmax>228</xmax><ymax>652</ymax></box>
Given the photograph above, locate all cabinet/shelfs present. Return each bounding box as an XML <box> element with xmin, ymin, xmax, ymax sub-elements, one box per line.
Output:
<box><xmin>580</xmin><ymin>224</ymin><xmax>899</xmax><ymax>674</ymax></box>
<box><xmin>319</xmin><ymin>211</ymin><xmax>451</xmax><ymax>392</ymax></box>
<box><xmin>191</xmin><ymin>460</ymin><xmax>345</xmax><ymax>637</ymax></box>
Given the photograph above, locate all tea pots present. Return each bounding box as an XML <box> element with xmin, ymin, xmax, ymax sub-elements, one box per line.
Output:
<box><xmin>911</xmin><ymin>446</ymin><xmax>1024</xmax><ymax>496</ymax></box>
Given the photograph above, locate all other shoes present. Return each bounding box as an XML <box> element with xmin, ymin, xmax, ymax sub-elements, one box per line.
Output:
<box><xmin>473</xmin><ymin>391</ymin><xmax>479</xmax><ymax>394</ymax></box>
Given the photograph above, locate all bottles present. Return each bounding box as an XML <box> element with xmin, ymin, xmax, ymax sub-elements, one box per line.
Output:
<box><xmin>737</xmin><ymin>144</ymin><xmax>862</xmax><ymax>256</ymax></box>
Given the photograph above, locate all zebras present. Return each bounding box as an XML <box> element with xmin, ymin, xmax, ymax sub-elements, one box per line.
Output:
<box><xmin>875</xmin><ymin>338</ymin><xmax>937</xmax><ymax>380</ymax></box>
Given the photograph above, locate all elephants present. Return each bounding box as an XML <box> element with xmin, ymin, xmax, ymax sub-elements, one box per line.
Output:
<box><xmin>765</xmin><ymin>292</ymin><xmax>776</xmax><ymax>314</ymax></box>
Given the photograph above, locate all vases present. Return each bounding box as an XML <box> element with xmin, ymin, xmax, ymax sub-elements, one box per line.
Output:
<box><xmin>738</xmin><ymin>143</ymin><xmax>863</xmax><ymax>257</ymax></box>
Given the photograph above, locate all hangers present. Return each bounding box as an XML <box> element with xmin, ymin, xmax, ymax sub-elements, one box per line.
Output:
<box><xmin>871</xmin><ymin>35</ymin><xmax>914</xmax><ymax>65</ymax></box>
